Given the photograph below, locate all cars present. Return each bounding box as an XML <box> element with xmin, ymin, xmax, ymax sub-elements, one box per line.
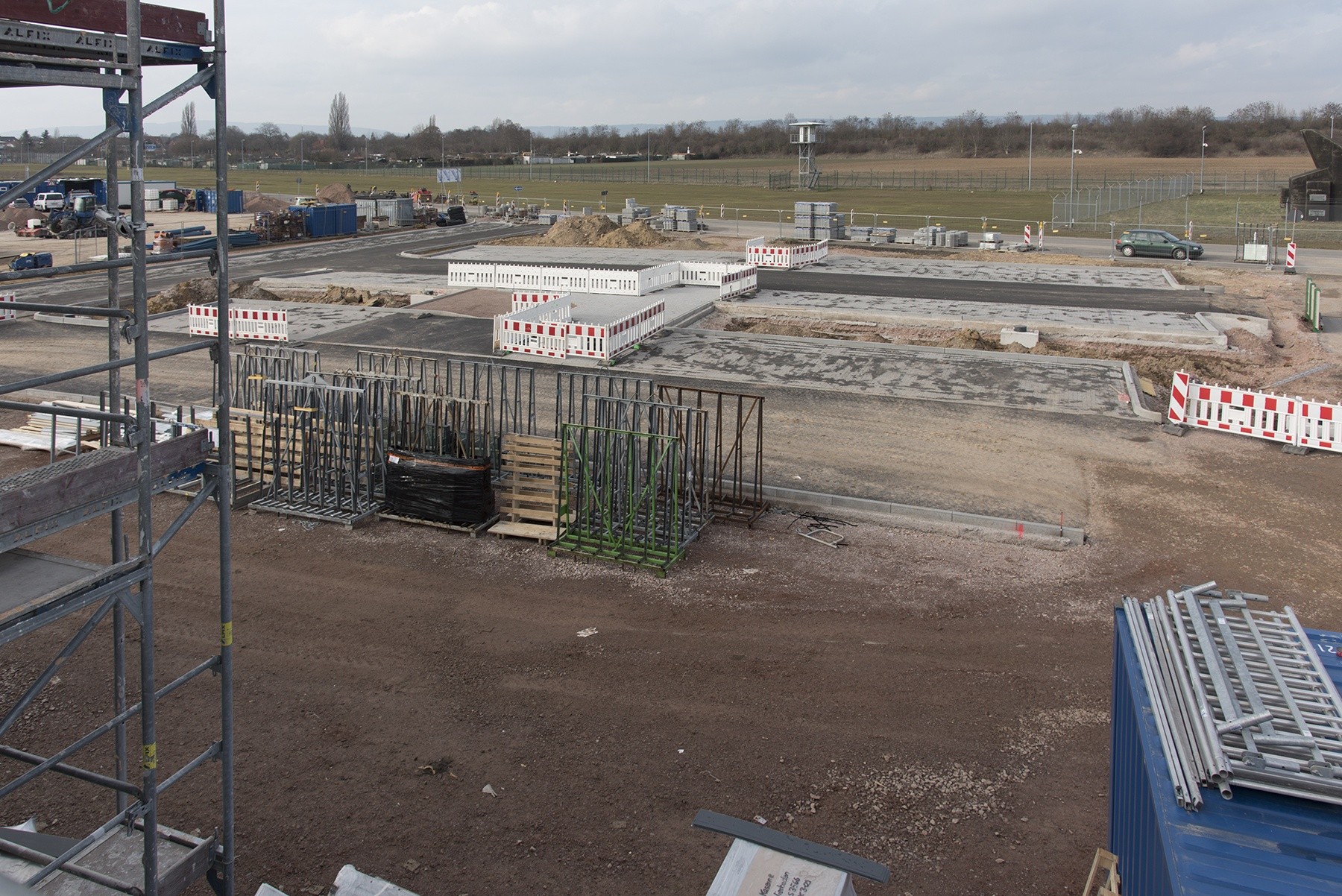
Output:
<box><xmin>7</xmin><ymin>198</ymin><xmax>30</xmax><ymax>209</ymax></box>
<box><xmin>159</xmin><ymin>189</ymin><xmax>188</xmax><ymax>209</ymax></box>
<box><xmin>65</xmin><ymin>189</ymin><xmax>96</xmax><ymax>211</ymax></box>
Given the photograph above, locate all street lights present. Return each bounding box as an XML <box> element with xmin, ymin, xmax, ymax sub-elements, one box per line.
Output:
<box><xmin>442</xmin><ymin>136</ymin><xmax>446</xmax><ymax>169</ymax></box>
<box><xmin>190</xmin><ymin>140</ymin><xmax>194</xmax><ymax>169</ymax></box>
<box><xmin>144</xmin><ymin>141</ymin><xmax>148</xmax><ymax>168</ymax></box>
<box><xmin>647</xmin><ymin>129</ymin><xmax>652</xmax><ymax>183</ymax></box>
<box><xmin>301</xmin><ymin>138</ymin><xmax>305</xmax><ymax>171</ymax></box>
<box><xmin>62</xmin><ymin>141</ymin><xmax>66</xmax><ymax>155</ymax></box>
<box><xmin>1069</xmin><ymin>123</ymin><xmax>1079</xmax><ymax>228</ymax></box>
<box><xmin>241</xmin><ymin>139</ymin><xmax>245</xmax><ymax>172</ymax></box>
<box><xmin>21</xmin><ymin>142</ymin><xmax>24</xmax><ymax>164</ymax></box>
<box><xmin>529</xmin><ymin>132</ymin><xmax>535</xmax><ymax>180</ymax></box>
<box><xmin>365</xmin><ymin>137</ymin><xmax>368</xmax><ymax>171</ymax></box>
<box><xmin>27</xmin><ymin>142</ymin><xmax>31</xmax><ymax>163</ymax></box>
<box><xmin>1199</xmin><ymin>125</ymin><xmax>1208</xmax><ymax>194</ymax></box>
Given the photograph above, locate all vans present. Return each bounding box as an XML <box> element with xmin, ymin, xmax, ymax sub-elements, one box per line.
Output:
<box><xmin>32</xmin><ymin>192</ymin><xmax>65</xmax><ymax>212</ymax></box>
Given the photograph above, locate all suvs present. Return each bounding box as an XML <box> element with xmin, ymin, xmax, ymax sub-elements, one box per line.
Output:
<box><xmin>1115</xmin><ymin>229</ymin><xmax>1204</xmax><ymax>260</ymax></box>
